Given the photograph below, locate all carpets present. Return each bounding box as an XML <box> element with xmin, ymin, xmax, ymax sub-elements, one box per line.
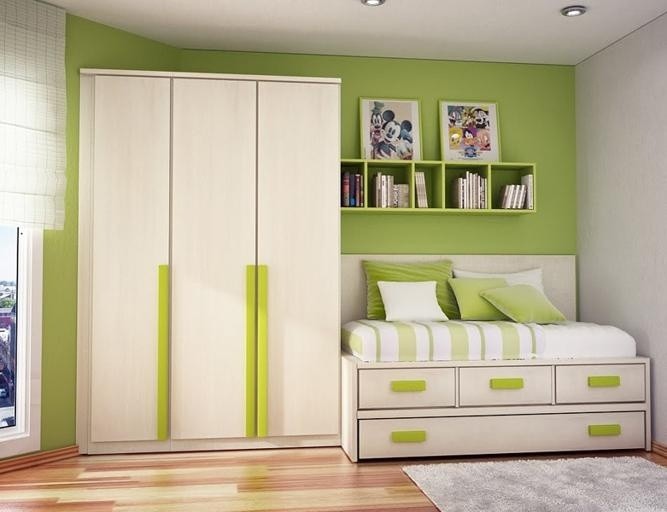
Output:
<box><xmin>397</xmin><ymin>454</ymin><xmax>667</xmax><ymax>512</ymax></box>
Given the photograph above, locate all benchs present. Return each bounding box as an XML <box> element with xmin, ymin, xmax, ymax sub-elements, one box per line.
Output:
<box><xmin>336</xmin><ymin>251</ymin><xmax>642</xmax><ymax>365</ymax></box>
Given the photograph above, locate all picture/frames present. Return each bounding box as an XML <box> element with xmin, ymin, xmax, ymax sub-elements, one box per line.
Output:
<box><xmin>356</xmin><ymin>96</ymin><xmax>426</xmax><ymax>162</ymax></box>
<box><xmin>437</xmin><ymin>97</ymin><xmax>504</xmax><ymax>164</ymax></box>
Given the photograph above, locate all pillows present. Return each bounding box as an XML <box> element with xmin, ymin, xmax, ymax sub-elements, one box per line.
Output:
<box><xmin>453</xmin><ymin>267</ymin><xmax>545</xmax><ymax>296</ymax></box>
<box><xmin>447</xmin><ymin>276</ymin><xmax>513</xmax><ymax>322</ymax></box>
<box><xmin>478</xmin><ymin>284</ymin><xmax>568</xmax><ymax>327</ymax></box>
<box><xmin>358</xmin><ymin>257</ymin><xmax>461</xmax><ymax>323</ymax></box>
<box><xmin>376</xmin><ymin>277</ymin><xmax>449</xmax><ymax>324</ymax></box>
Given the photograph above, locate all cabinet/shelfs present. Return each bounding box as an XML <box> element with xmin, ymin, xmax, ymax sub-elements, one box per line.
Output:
<box><xmin>76</xmin><ymin>63</ymin><xmax>344</xmax><ymax>452</ymax></box>
<box><xmin>338</xmin><ymin>158</ymin><xmax>539</xmax><ymax>218</ymax></box>
<box><xmin>338</xmin><ymin>350</ymin><xmax>655</xmax><ymax>462</ymax></box>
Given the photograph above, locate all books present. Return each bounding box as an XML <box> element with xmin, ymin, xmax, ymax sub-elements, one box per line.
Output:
<box><xmin>341</xmin><ymin>163</ymin><xmax>533</xmax><ymax>211</ymax></box>
